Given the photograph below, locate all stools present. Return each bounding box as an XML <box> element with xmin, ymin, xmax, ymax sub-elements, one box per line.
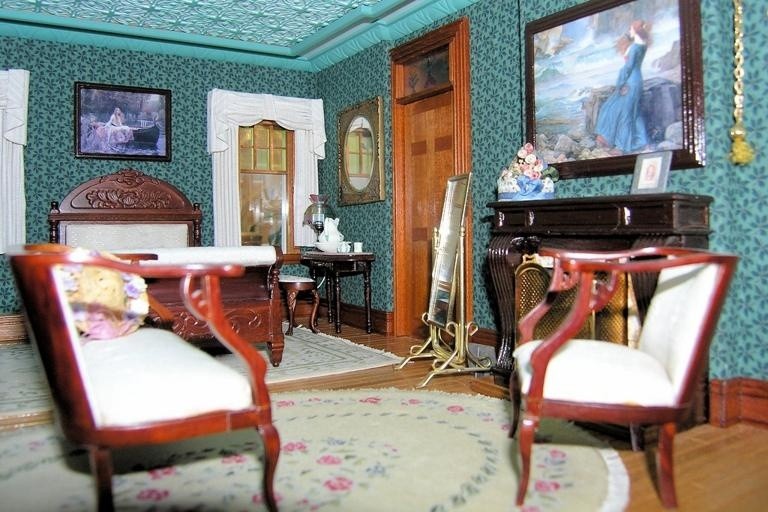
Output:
<box><xmin>276</xmin><ymin>273</ymin><xmax>321</xmax><ymax>336</ymax></box>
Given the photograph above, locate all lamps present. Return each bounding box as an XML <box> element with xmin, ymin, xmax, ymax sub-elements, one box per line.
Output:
<box><xmin>302</xmin><ymin>193</ymin><xmax>335</xmax><ymax>251</ymax></box>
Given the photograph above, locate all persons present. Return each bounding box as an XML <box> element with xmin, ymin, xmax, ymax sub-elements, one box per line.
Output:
<box><xmin>103</xmin><ymin>107</ymin><xmax>126</xmax><ymax>129</ymax></box>
<box><xmin>594</xmin><ymin>19</ymin><xmax>654</xmax><ymax>153</ymax></box>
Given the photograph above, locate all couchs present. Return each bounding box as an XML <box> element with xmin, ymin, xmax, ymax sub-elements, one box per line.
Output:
<box><xmin>5</xmin><ymin>243</ymin><xmax>282</xmax><ymax>511</ymax></box>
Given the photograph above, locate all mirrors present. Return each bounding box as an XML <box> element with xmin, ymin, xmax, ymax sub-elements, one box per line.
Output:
<box><xmin>426</xmin><ymin>171</ymin><xmax>473</xmax><ymax>332</ymax></box>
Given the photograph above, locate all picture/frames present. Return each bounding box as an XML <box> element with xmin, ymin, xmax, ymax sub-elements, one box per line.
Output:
<box><xmin>337</xmin><ymin>95</ymin><xmax>385</xmax><ymax>208</ymax></box>
<box><xmin>630</xmin><ymin>150</ymin><xmax>674</xmax><ymax>195</ymax></box>
<box><xmin>72</xmin><ymin>80</ymin><xmax>173</xmax><ymax>163</ymax></box>
<box><xmin>524</xmin><ymin>0</ymin><xmax>706</xmax><ymax>181</ymax></box>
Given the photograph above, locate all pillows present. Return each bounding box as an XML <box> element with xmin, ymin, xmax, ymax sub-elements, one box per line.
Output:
<box><xmin>52</xmin><ymin>246</ymin><xmax>149</xmax><ymax>341</ymax></box>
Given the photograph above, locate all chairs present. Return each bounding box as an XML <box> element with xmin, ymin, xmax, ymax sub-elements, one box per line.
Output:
<box><xmin>507</xmin><ymin>244</ymin><xmax>740</xmax><ymax>510</ymax></box>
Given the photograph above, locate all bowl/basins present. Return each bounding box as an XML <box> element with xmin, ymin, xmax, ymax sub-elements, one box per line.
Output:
<box><xmin>314</xmin><ymin>240</ymin><xmax>352</xmax><ymax>255</ymax></box>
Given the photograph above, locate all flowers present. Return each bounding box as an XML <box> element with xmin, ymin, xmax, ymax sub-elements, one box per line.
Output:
<box><xmin>500</xmin><ymin>142</ymin><xmax>543</xmax><ymax>179</ymax></box>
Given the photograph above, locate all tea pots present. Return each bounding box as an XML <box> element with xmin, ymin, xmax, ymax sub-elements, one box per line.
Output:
<box><xmin>318</xmin><ymin>217</ymin><xmax>344</xmax><ymax>242</ymax></box>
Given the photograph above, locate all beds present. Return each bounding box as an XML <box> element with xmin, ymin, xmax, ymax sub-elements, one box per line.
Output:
<box><xmin>47</xmin><ymin>168</ymin><xmax>284</xmax><ymax>368</ymax></box>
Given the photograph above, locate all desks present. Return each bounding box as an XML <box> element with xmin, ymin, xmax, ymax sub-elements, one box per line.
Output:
<box><xmin>299</xmin><ymin>251</ymin><xmax>375</xmax><ymax>334</ymax></box>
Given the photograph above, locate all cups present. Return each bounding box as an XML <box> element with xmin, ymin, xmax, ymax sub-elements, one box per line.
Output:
<box><xmin>353</xmin><ymin>242</ymin><xmax>363</xmax><ymax>253</ymax></box>
